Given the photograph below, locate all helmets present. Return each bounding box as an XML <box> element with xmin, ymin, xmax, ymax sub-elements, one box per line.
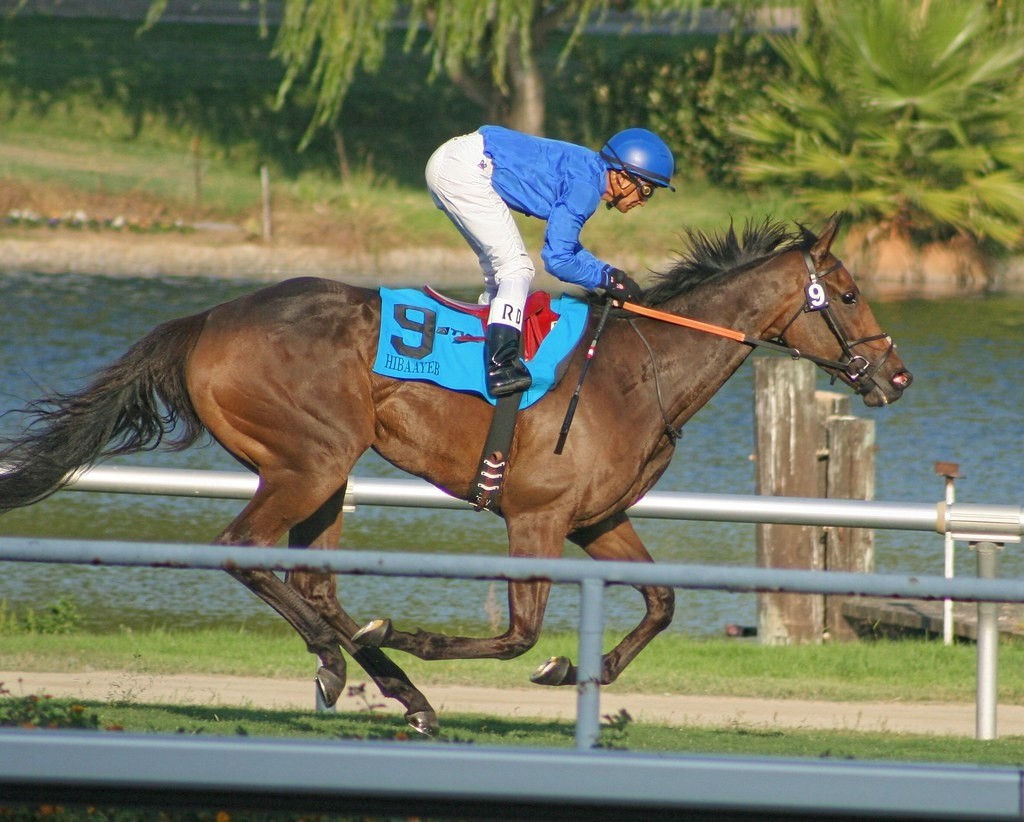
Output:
<box><xmin>600</xmin><ymin>127</ymin><xmax>677</xmax><ymax>193</ymax></box>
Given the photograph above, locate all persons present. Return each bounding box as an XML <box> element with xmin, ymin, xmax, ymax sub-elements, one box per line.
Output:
<box><xmin>425</xmin><ymin>127</ymin><xmax>676</xmax><ymax>398</ymax></box>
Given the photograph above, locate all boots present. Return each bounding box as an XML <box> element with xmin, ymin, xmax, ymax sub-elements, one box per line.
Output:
<box><xmin>483</xmin><ymin>323</ymin><xmax>532</xmax><ymax>398</ymax></box>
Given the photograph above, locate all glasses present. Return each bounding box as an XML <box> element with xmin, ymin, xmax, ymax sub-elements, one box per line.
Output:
<box><xmin>617</xmin><ymin>170</ymin><xmax>656</xmax><ymax>202</ymax></box>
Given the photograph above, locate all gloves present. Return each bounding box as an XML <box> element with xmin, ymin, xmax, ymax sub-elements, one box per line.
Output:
<box><xmin>604</xmin><ymin>268</ymin><xmax>642</xmax><ymax>308</ymax></box>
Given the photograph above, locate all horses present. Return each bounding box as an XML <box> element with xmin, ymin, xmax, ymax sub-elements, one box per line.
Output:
<box><xmin>0</xmin><ymin>210</ymin><xmax>912</xmax><ymax>742</ymax></box>
